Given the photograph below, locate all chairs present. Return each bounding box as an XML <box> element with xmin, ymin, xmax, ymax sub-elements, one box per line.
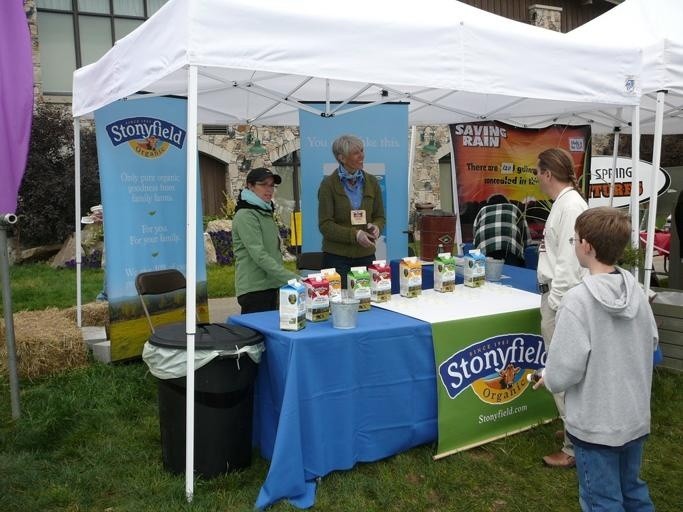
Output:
<box><xmin>135</xmin><ymin>267</ymin><xmax>201</xmax><ymax>336</ymax></box>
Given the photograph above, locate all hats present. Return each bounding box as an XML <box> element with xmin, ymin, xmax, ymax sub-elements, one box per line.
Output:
<box><xmin>244</xmin><ymin>167</ymin><xmax>282</xmax><ymax>185</ymax></box>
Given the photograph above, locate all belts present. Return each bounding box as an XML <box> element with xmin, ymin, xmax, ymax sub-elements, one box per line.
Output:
<box><xmin>535</xmin><ymin>281</ymin><xmax>550</xmax><ymax>295</ymax></box>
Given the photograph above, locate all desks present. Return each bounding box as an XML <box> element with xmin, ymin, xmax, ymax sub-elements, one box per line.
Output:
<box><xmin>639</xmin><ymin>230</ymin><xmax>669</xmax><ymax>278</ymax></box>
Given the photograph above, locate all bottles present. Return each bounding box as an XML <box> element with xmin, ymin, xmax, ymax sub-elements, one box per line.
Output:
<box><xmin>436</xmin><ymin>244</ymin><xmax>444</xmax><ymax>255</ymax></box>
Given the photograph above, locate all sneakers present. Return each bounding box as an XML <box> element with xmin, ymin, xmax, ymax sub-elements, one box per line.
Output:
<box><xmin>553</xmin><ymin>428</ymin><xmax>565</xmax><ymax>440</ymax></box>
<box><xmin>540</xmin><ymin>450</ymin><xmax>577</xmax><ymax>471</ymax></box>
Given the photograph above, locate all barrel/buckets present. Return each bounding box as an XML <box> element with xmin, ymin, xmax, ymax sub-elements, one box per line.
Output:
<box><xmin>419</xmin><ymin>215</ymin><xmax>457</xmax><ymax>261</ymax></box>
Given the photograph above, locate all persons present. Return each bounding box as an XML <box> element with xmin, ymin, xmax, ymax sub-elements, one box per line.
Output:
<box><xmin>534</xmin><ymin>147</ymin><xmax>592</xmax><ymax>469</ymax></box>
<box><xmin>230</xmin><ymin>166</ymin><xmax>305</xmax><ymax>315</ymax></box>
<box><xmin>469</xmin><ymin>193</ymin><xmax>532</xmax><ymax>268</ymax></box>
<box><xmin>316</xmin><ymin>134</ymin><xmax>385</xmax><ymax>289</ymax></box>
<box><xmin>531</xmin><ymin>204</ymin><xmax>662</xmax><ymax>511</ymax></box>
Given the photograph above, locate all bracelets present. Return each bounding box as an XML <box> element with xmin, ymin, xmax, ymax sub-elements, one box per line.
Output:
<box><xmin>355</xmin><ymin>229</ymin><xmax>362</xmax><ymax>243</ymax></box>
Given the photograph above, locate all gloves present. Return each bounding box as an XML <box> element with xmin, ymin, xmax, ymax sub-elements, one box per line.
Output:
<box><xmin>355</xmin><ymin>222</ymin><xmax>380</xmax><ymax>250</ymax></box>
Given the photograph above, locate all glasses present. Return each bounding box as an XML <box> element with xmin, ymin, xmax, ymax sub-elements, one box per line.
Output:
<box><xmin>531</xmin><ymin>168</ymin><xmax>541</xmax><ymax>175</ymax></box>
<box><xmin>254</xmin><ymin>182</ymin><xmax>275</xmax><ymax>188</ymax></box>
<box><xmin>567</xmin><ymin>237</ymin><xmax>581</xmax><ymax>244</ymax></box>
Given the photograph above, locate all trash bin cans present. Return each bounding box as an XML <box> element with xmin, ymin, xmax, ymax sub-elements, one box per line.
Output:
<box><xmin>149</xmin><ymin>322</ymin><xmax>265</xmax><ymax>481</ymax></box>
